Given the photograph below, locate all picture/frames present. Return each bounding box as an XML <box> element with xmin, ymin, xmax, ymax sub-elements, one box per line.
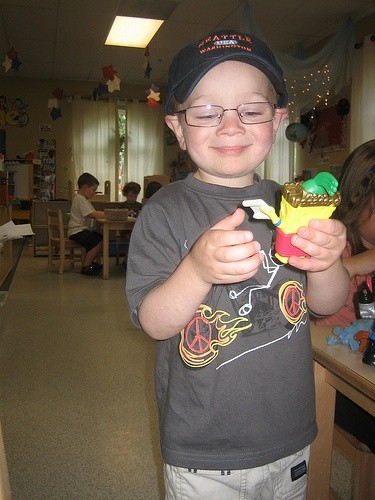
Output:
<box><xmin>307</xmin><ymin>102</ymin><xmax>347</xmax><ymax>154</ymax></box>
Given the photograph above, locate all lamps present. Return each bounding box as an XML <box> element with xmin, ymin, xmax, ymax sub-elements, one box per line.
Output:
<box><xmin>286</xmin><ymin>107</ymin><xmax>318</xmax><ymax>143</ymax></box>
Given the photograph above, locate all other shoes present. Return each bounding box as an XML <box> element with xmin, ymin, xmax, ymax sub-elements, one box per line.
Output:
<box><xmin>81</xmin><ymin>262</ymin><xmax>101</xmax><ymax>276</ymax></box>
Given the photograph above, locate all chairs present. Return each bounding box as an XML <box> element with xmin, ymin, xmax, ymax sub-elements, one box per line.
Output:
<box><xmin>46</xmin><ymin>208</ymin><xmax>87</xmax><ymax>273</ymax></box>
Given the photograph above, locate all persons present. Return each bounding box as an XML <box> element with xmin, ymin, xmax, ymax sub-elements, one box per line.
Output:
<box><xmin>125</xmin><ymin>30</ymin><xmax>351</xmax><ymax>500</ymax></box>
<box><xmin>142</xmin><ymin>181</ymin><xmax>162</xmax><ymax>203</ymax></box>
<box><xmin>310</xmin><ymin>139</ymin><xmax>375</xmax><ymax>326</ymax></box>
<box><xmin>118</xmin><ymin>182</ymin><xmax>144</xmax><ymax>266</ymax></box>
<box><xmin>67</xmin><ymin>173</ymin><xmax>105</xmax><ymax>276</ymax></box>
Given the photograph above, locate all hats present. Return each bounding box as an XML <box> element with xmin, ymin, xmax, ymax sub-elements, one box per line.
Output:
<box><xmin>164</xmin><ymin>29</ymin><xmax>289</xmax><ymax>112</ymax></box>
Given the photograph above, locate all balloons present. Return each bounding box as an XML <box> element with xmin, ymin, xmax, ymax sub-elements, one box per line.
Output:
<box><xmin>286</xmin><ymin>123</ymin><xmax>307</xmax><ymax>143</ymax></box>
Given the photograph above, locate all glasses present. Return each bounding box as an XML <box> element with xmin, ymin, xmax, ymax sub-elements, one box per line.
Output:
<box><xmin>173</xmin><ymin>102</ymin><xmax>278</xmax><ymax>127</ymax></box>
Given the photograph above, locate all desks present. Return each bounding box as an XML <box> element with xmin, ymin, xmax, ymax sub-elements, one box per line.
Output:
<box><xmin>304</xmin><ymin>325</ymin><xmax>375</xmax><ymax>500</ymax></box>
<box><xmin>95</xmin><ymin>218</ymin><xmax>138</xmax><ymax>280</ymax></box>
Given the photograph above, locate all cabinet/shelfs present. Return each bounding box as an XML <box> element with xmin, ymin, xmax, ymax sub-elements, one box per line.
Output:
<box><xmin>0</xmin><ymin>170</ymin><xmax>13</xmax><ymax>288</ymax></box>
<box><xmin>32</xmin><ymin>201</ymin><xmax>124</xmax><ymax>256</ymax></box>
<box><xmin>5</xmin><ymin>159</ymin><xmax>33</xmax><ymax>221</ymax></box>
